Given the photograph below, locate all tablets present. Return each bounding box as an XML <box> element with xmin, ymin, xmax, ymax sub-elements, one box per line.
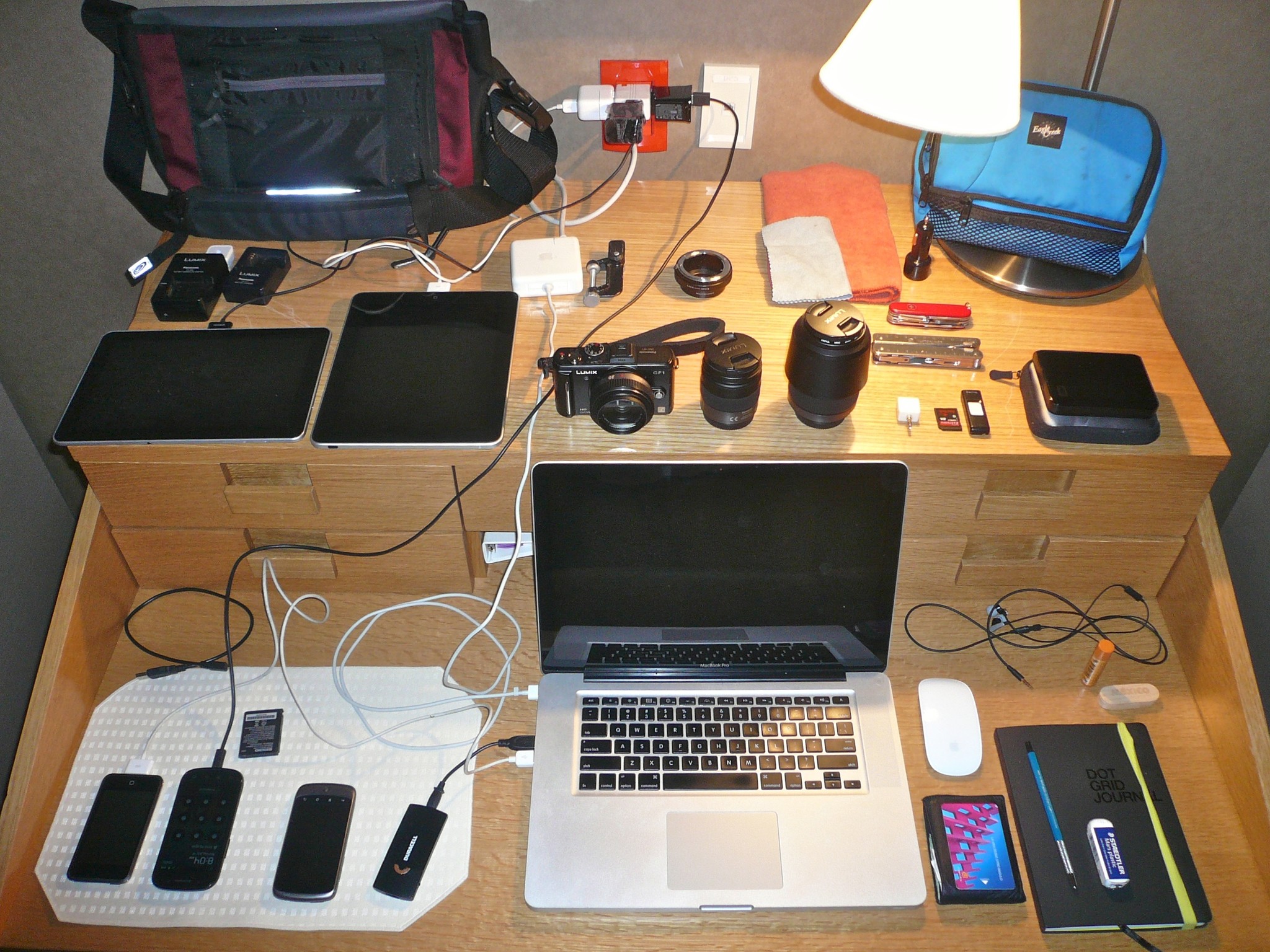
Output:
<box><xmin>52</xmin><ymin>326</ymin><xmax>333</xmax><ymax>444</ymax></box>
<box><xmin>309</xmin><ymin>285</ymin><xmax>520</xmax><ymax>448</ymax></box>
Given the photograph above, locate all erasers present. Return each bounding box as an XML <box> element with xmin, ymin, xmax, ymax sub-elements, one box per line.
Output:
<box><xmin>1086</xmin><ymin>818</ymin><xmax>1130</xmax><ymax>889</ymax></box>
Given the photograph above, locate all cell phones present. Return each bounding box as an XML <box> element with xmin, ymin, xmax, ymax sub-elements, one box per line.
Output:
<box><xmin>272</xmin><ymin>781</ymin><xmax>356</xmax><ymax>902</ymax></box>
<box><xmin>66</xmin><ymin>773</ymin><xmax>164</xmax><ymax>885</ymax></box>
<box><xmin>151</xmin><ymin>767</ymin><xmax>244</xmax><ymax>892</ymax></box>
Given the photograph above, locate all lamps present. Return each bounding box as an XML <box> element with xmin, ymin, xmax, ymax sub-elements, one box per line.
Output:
<box><xmin>805</xmin><ymin>0</ymin><xmax>1028</xmax><ymax>284</ymax></box>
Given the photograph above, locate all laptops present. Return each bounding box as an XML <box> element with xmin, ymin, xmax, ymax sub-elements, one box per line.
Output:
<box><xmin>543</xmin><ymin>624</ymin><xmax>885</xmax><ymax>670</ymax></box>
<box><xmin>526</xmin><ymin>459</ymin><xmax>930</xmax><ymax>910</ymax></box>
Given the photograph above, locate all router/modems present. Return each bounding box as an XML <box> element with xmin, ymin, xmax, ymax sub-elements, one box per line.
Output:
<box><xmin>373</xmin><ymin>804</ymin><xmax>448</xmax><ymax>901</ymax></box>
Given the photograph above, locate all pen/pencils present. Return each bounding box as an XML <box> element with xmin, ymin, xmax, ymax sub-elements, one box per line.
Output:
<box><xmin>1025</xmin><ymin>741</ymin><xmax>1077</xmax><ymax>887</ymax></box>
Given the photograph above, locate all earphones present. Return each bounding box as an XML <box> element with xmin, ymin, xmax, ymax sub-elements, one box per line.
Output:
<box><xmin>987</xmin><ymin>604</ymin><xmax>1008</xmax><ymax>617</ymax></box>
<box><xmin>990</xmin><ymin>614</ymin><xmax>1007</xmax><ymax>626</ymax></box>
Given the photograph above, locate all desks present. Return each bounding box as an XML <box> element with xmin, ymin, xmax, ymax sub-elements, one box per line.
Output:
<box><xmin>0</xmin><ymin>175</ymin><xmax>1265</xmax><ymax>952</ymax></box>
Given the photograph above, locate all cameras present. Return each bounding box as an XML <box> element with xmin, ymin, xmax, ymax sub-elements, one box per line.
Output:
<box><xmin>537</xmin><ymin>342</ymin><xmax>678</xmax><ymax>436</ymax></box>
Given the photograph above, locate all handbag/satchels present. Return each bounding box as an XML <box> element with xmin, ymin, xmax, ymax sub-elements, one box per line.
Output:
<box><xmin>119</xmin><ymin>3</ymin><xmax>497</xmax><ymax>202</ymax></box>
<box><xmin>911</xmin><ymin>78</ymin><xmax>1165</xmax><ymax>277</ymax></box>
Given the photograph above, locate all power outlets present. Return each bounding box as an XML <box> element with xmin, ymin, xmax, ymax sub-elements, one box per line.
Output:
<box><xmin>599</xmin><ymin>62</ymin><xmax>674</xmax><ymax>155</ymax></box>
<box><xmin>696</xmin><ymin>62</ymin><xmax>762</xmax><ymax>153</ymax></box>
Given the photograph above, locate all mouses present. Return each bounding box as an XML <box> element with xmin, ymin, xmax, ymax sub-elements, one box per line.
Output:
<box><xmin>919</xmin><ymin>677</ymin><xmax>984</xmax><ymax>776</ymax></box>
